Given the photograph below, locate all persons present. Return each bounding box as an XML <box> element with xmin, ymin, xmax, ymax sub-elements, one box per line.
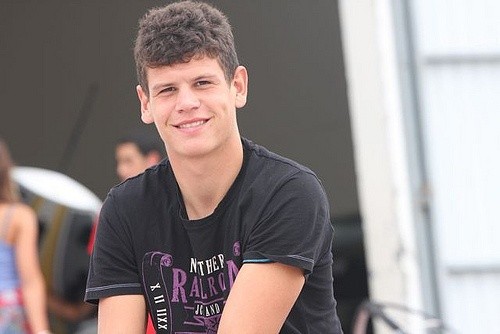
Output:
<box><xmin>84</xmin><ymin>2</ymin><xmax>343</xmax><ymax>334</ymax></box>
<box><xmin>0</xmin><ymin>139</ymin><xmax>51</xmax><ymax>334</ymax></box>
<box><xmin>87</xmin><ymin>137</ymin><xmax>165</xmax><ymax>334</ymax></box>
